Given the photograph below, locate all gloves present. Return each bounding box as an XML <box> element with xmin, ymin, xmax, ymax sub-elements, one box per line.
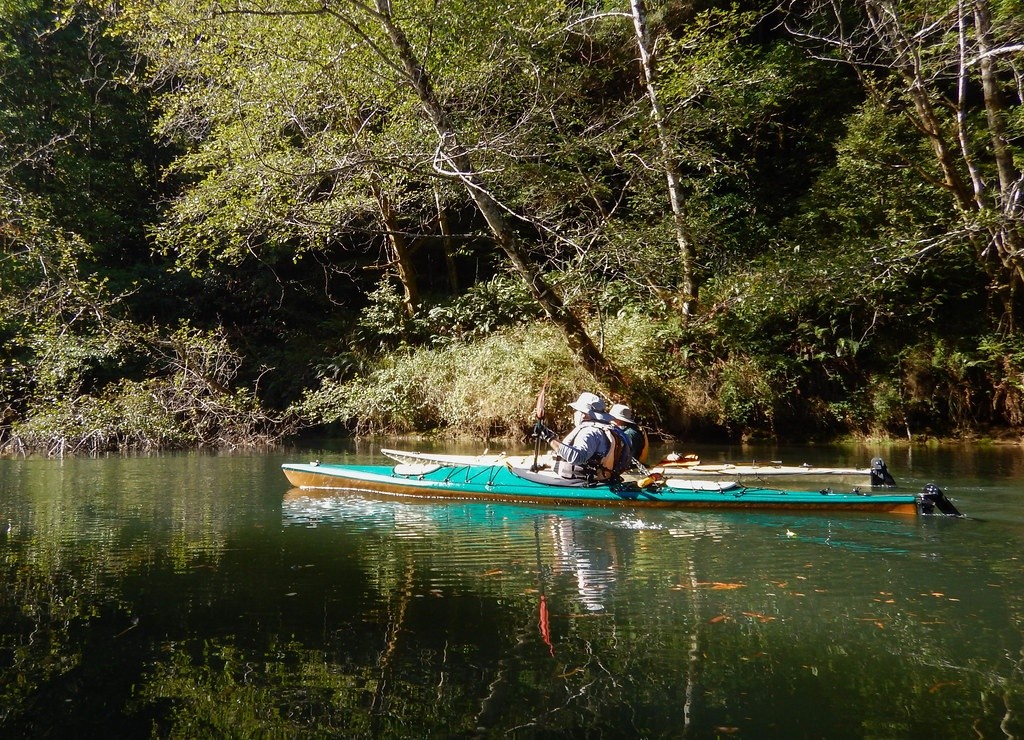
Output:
<box><xmin>534</xmin><ymin>425</ymin><xmax>556</xmax><ymax>444</ymax></box>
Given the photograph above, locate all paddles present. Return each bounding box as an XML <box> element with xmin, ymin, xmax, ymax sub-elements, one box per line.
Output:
<box><xmin>531</xmin><ymin>374</ymin><xmax>549</xmax><ymax>470</ymax></box>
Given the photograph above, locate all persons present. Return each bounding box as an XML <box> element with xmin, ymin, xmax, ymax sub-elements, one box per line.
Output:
<box><xmin>532</xmin><ymin>392</ymin><xmax>614</xmax><ymax>479</ymax></box>
<box><xmin>609</xmin><ymin>404</ymin><xmax>645</xmax><ymax>470</ymax></box>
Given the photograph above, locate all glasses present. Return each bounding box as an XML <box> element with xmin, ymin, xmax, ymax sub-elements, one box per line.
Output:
<box><xmin>571</xmin><ymin>408</ymin><xmax>577</xmax><ymax>413</ymax></box>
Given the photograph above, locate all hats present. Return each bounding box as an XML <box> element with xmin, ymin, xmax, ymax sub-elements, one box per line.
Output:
<box><xmin>567</xmin><ymin>392</ymin><xmax>615</xmax><ymax>421</ymax></box>
<box><xmin>608</xmin><ymin>404</ymin><xmax>637</xmax><ymax>425</ymax></box>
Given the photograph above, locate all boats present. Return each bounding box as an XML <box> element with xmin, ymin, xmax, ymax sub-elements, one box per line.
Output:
<box><xmin>379</xmin><ymin>447</ymin><xmax>897</xmax><ymax>490</ymax></box>
<box><xmin>281</xmin><ymin>460</ymin><xmax>963</xmax><ymax>517</ymax></box>
